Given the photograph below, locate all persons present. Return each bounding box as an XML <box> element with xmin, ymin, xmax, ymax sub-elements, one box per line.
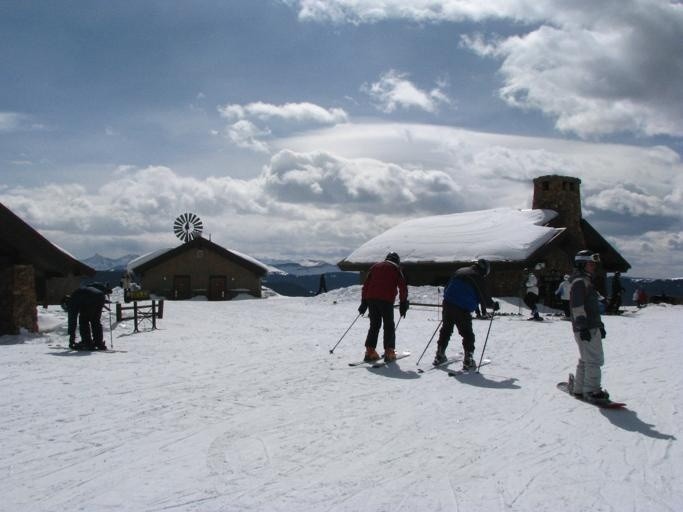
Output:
<box><xmin>521</xmin><ymin>267</ymin><xmax>543</xmax><ymax>321</ymax></box>
<box><xmin>568</xmin><ymin>249</ymin><xmax>609</xmax><ymax>400</ymax></box>
<box><xmin>555</xmin><ymin>272</ymin><xmax>571</xmax><ymax>318</ymax></box>
<box><xmin>357</xmin><ymin>251</ymin><xmax>408</xmax><ymax>361</ymax></box>
<box><xmin>61</xmin><ymin>287</ymin><xmax>108</xmax><ymax>352</ymax></box>
<box><xmin>604</xmin><ymin>269</ymin><xmax>625</xmax><ymax>315</ymax></box>
<box><xmin>631</xmin><ymin>289</ymin><xmax>640</xmax><ymax>305</ymax></box>
<box><xmin>79</xmin><ymin>283</ymin><xmax>112</xmax><ymax>312</ymax></box>
<box><xmin>429</xmin><ymin>261</ymin><xmax>500</xmax><ymax>371</ymax></box>
<box><xmin>315</xmin><ymin>271</ymin><xmax>327</xmax><ymax>295</ymax></box>
<box><xmin>639</xmin><ymin>287</ymin><xmax>646</xmax><ymax>303</ymax></box>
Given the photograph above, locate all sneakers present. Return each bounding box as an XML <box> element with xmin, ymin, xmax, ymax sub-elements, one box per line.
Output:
<box><xmin>383</xmin><ymin>347</ymin><xmax>397</xmax><ymax>362</ymax></box>
<box><xmin>77</xmin><ymin>338</ymin><xmax>105</xmax><ymax>348</ymax></box>
<box><xmin>363</xmin><ymin>344</ymin><xmax>380</xmax><ymax>361</ymax></box>
<box><xmin>574</xmin><ymin>385</ymin><xmax>610</xmax><ymax>403</ymax></box>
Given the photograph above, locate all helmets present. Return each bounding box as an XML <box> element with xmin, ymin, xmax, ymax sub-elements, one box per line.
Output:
<box><xmin>573</xmin><ymin>249</ymin><xmax>601</xmax><ymax>264</ymax></box>
<box><xmin>563</xmin><ymin>275</ymin><xmax>570</xmax><ymax>280</ymax></box>
<box><xmin>477</xmin><ymin>257</ymin><xmax>491</xmax><ymax>281</ymax></box>
<box><xmin>383</xmin><ymin>251</ymin><xmax>400</xmax><ymax>265</ymax></box>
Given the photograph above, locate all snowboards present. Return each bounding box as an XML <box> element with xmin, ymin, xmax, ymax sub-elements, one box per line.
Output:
<box><xmin>558</xmin><ymin>379</ymin><xmax>627</xmax><ymax>410</ymax></box>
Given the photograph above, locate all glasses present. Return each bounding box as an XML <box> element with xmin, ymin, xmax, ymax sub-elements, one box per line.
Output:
<box><xmin>575</xmin><ymin>253</ymin><xmax>600</xmax><ymax>262</ymax></box>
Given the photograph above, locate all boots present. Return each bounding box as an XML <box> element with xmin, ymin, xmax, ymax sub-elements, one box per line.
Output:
<box><xmin>461</xmin><ymin>347</ymin><xmax>477</xmax><ymax>370</ymax></box>
<box><xmin>431</xmin><ymin>343</ymin><xmax>448</xmax><ymax>365</ymax></box>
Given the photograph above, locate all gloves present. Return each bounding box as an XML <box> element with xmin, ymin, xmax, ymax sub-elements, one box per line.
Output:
<box><xmin>357</xmin><ymin>301</ymin><xmax>368</xmax><ymax>316</ymax></box>
<box><xmin>399</xmin><ymin>303</ymin><xmax>409</xmax><ymax>318</ymax></box>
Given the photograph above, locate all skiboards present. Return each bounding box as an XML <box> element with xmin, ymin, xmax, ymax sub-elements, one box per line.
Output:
<box><xmin>348</xmin><ymin>350</ymin><xmax>409</xmax><ymax>368</ymax></box>
<box><xmin>418</xmin><ymin>352</ymin><xmax>492</xmax><ymax>375</ymax></box>
<box><xmin>508</xmin><ymin>315</ymin><xmax>553</xmax><ymax>323</ymax></box>
<box><xmin>48</xmin><ymin>343</ymin><xmax>128</xmax><ymax>354</ymax></box>
